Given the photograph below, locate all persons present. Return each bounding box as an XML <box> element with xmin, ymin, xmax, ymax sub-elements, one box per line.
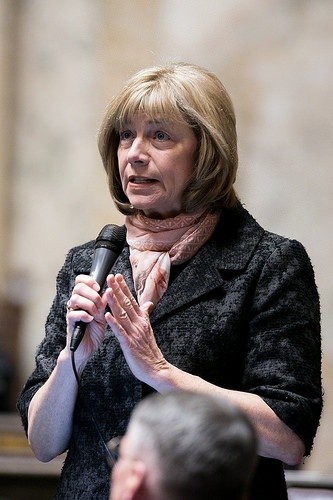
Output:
<box><xmin>16</xmin><ymin>57</ymin><xmax>323</xmax><ymax>500</ymax></box>
<box><xmin>100</xmin><ymin>389</ymin><xmax>259</xmax><ymax>500</ymax></box>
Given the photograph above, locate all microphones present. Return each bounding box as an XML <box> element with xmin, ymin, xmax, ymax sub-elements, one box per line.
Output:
<box><xmin>69</xmin><ymin>224</ymin><xmax>127</xmax><ymax>351</ymax></box>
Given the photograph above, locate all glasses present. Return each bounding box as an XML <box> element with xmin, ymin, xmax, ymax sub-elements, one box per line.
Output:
<box><xmin>104</xmin><ymin>437</ymin><xmax>131</xmax><ymax>468</ymax></box>
